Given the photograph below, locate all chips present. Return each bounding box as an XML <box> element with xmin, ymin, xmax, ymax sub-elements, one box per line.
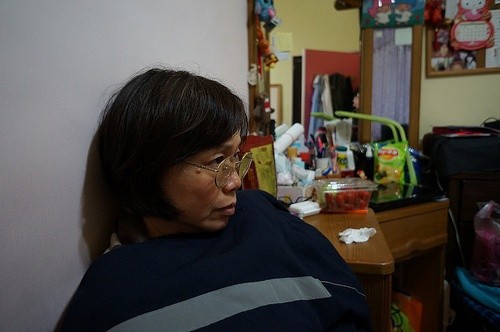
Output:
<box><xmin>375</xmin><ymin>142</ymin><xmax>407</xmax><ymax>201</ymax></box>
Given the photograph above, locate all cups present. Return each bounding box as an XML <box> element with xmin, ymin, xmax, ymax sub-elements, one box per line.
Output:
<box><xmin>315</xmin><ymin>158</ymin><xmax>329</xmax><ymax>171</ymax></box>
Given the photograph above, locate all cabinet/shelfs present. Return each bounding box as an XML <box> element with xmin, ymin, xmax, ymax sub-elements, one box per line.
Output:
<box><xmin>282</xmin><ymin>197</ymin><xmax>450</xmax><ymax>332</ymax></box>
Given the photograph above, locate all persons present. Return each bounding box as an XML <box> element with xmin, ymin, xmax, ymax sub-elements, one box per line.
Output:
<box><xmin>432</xmin><ymin>31</ymin><xmax>466</xmax><ymax>72</ymax></box>
<box><xmin>63</xmin><ymin>68</ymin><xmax>379</xmax><ymax>332</ymax></box>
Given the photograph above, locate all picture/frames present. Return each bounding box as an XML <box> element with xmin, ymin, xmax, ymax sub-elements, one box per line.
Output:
<box><xmin>426</xmin><ymin>0</ymin><xmax>500</xmax><ymax>78</ymax></box>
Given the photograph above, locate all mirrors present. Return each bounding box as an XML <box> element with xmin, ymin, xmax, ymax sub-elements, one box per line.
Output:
<box><xmin>270</xmin><ymin>0</ymin><xmax>361</xmax><ymax>142</ymax></box>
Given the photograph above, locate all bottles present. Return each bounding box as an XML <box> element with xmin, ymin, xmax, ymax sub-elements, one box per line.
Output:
<box><xmin>333</xmin><ymin>146</ymin><xmax>348</xmax><ymax>171</ymax></box>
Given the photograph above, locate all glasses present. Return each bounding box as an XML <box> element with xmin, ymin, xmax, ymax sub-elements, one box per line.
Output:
<box><xmin>180</xmin><ymin>151</ymin><xmax>254</xmax><ymax>188</ymax></box>
